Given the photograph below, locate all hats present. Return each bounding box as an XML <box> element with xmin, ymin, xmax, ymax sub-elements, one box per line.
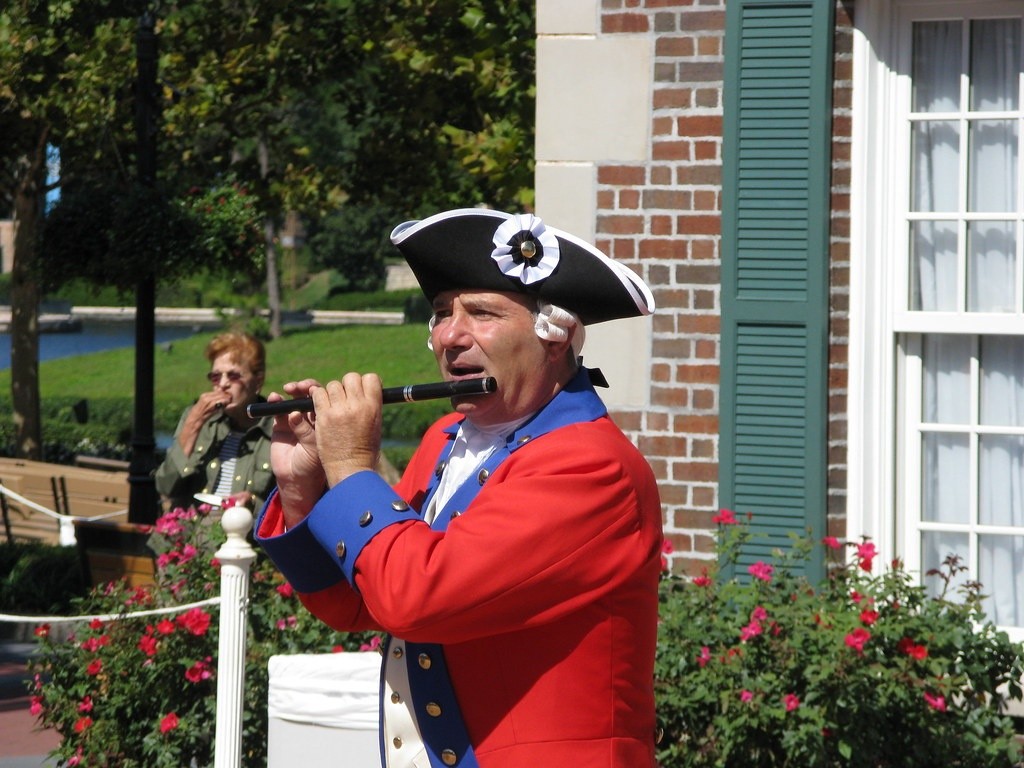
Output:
<box><xmin>389</xmin><ymin>207</ymin><xmax>656</xmax><ymax>325</ymax></box>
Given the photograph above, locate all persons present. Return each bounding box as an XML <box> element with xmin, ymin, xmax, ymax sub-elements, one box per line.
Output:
<box><xmin>248</xmin><ymin>206</ymin><xmax>667</xmax><ymax>768</ymax></box>
<box><xmin>155</xmin><ymin>329</ymin><xmax>282</xmax><ymax>516</ymax></box>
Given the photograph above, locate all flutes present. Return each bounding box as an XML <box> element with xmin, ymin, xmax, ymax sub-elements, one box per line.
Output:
<box><xmin>241</xmin><ymin>376</ymin><xmax>499</xmax><ymax>421</ymax></box>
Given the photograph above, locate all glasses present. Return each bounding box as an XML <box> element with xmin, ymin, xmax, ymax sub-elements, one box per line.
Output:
<box><xmin>208</xmin><ymin>371</ymin><xmax>251</xmax><ymax>383</ymax></box>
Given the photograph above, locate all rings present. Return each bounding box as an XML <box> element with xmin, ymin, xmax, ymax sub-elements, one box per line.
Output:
<box><xmin>215</xmin><ymin>400</ymin><xmax>221</xmax><ymax>408</ymax></box>
